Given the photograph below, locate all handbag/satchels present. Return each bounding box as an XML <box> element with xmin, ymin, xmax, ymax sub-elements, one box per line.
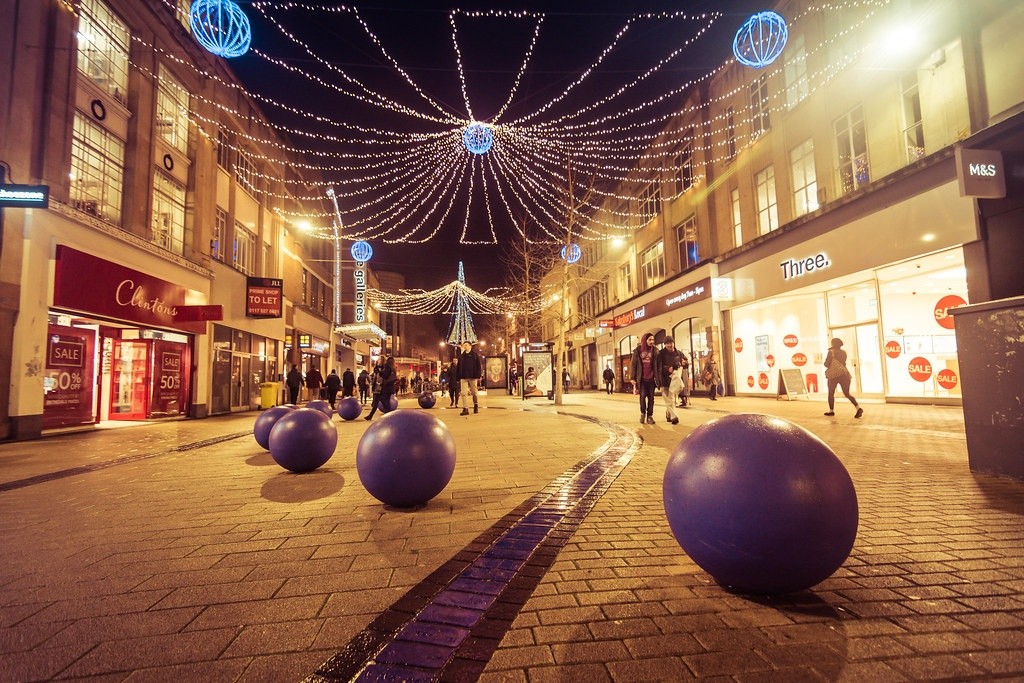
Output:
<box><xmin>717</xmin><ymin>382</ymin><xmax>724</xmax><ymax>395</ymax></box>
<box><xmin>825</xmin><ymin>348</ymin><xmax>847</xmax><ymax>380</ymax></box>
<box><xmin>669</xmin><ymin>366</ymin><xmax>684</xmax><ymax>393</ymax></box>
<box><xmin>704</xmin><ymin>371</ymin><xmax>712</xmax><ymax>380</ymax></box>
<box><xmin>566</xmin><ymin>372</ymin><xmax>570</xmax><ymax>382</ymax></box>
<box><xmin>365</xmin><ymin>375</ymin><xmax>370</xmax><ymax>386</ymax></box>
<box><xmin>382</xmin><ymin>367</ymin><xmax>398</xmax><ymax>385</ymax></box>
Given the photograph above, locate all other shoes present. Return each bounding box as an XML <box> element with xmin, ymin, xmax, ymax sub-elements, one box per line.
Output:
<box><xmin>680</xmin><ymin>403</ymin><xmax>686</xmax><ymax>406</ymax></box>
<box><xmin>474</xmin><ymin>404</ymin><xmax>479</xmax><ymax>413</ymax></box>
<box><xmin>824</xmin><ymin>411</ymin><xmax>835</xmax><ymax>416</ymax></box>
<box><xmin>455</xmin><ymin>404</ymin><xmax>458</xmax><ymax>408</ymax></box>
<box><xmin>364</xmin><ymin>415</ymin><xmax>372</xmax><ymax>420</ymax></box>
<box><xmin>450</xmin><ymin>401</ymin><xmax>454</xmax><ymax>406</ymax></box>
<box><xmin>710</xmin><ymin>396</ymin><xmax>716</xmax><ymax>400</ymax></box>
<box><xmin>854</xmin><ymin>407</ymin><xmax>863</xmax><ymax>418</ymax></box>
<box><xmin>672</xmin><ymin>418</ymin><xmax>679</xmax><ymax>424</ymax></box>
<box><xmin>460</xmin><ymin>408</ymin><xmax>469</xmax><ymax>415</ymax></box>
<box><xmin>667</xmin><ymin>416</ymin><xmax>673</xmax><ymax>422</ymax></box>
<box><xmin>640</xmin><ymin>412</ymin><xmax>645</xmax><ymax>423</ymax></box>
<box><xmin>647</xmin><ymin>415</ymin><xmax>655</xmax><ymax>424</ymax></box>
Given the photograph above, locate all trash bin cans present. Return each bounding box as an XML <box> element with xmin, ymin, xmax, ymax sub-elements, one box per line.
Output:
<box><xmin>257</xmin><ymin>382</ymin><xmax>283</xmax><ymax>411</ymax></box>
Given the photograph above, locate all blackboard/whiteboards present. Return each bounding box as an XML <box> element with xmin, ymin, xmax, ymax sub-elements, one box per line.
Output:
<box><xmin>777</xmin><ymin>368</ymin><xmax>808</xmax><ymax>397</ymax></box>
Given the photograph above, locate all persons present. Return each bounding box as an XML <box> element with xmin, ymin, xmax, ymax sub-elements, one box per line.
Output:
<box><xmin>343</xmin><ymin>368</ymin><xmax>357</xmax><ymax>397</ymax></box>
<box><xmin>456</xmin><ymin>341</ymin><xmax>482</xmax><ymax>416</ymax></box>
<box><xmin>629</xmin><ymin>333</ymin><xmax>685</xmax><ymax>424</ymax></box>
<box><xmin>824</xmin><ymin>338</ymin><xmax>863</xmax><ymax>418</ymax></box>
<box><xmin>358</xmin><ymin>370</ymin><xmax>371</xmax><ymax>403</ymax></box>
<box><xmin>562</xmin><ymin>369</ymin><xmax>571</xmax><ymax>394</ymax></box>
<box><xmin>396</xmin><ymin>375</ymin><xmax>409</xmax><ymax>393</ymax></box>
<box><xmin>322</xmin><ymin>369</ymin><xmax>340</xmax><ymax>410</ymax></box>
<box><xmin>416</xmin><ymin>373</ymin><xmax>428</xmax><ymax>382</ymax></box>
<box><xmin>487</xmin><ymin>358</ymin><xmax>506</xmax><ymax>382</ymax></box>
<box><xmin>446</xmin><ymin>358</ymin><xmax>461</xmax><ymax>408</ymax></box>
<box><xmin>677</xmin><ymin>350</ymin><xmax>690</xmax><ymax>407</ymax></box>
<box><xmin>602</xmin><ymin>365</ymin><xmax>615</xmax><ymax>395</ymax></box>
<box><xmin>704</xmin><ymin>354</ymin><xmax>721</xmax><ymax>400</ymax></box>
<box><xmin>439</xmin><ymin>367</ymin><xmax>448</xmax><ymax>397</ymax></box>
<box><xmin>364</xmin><ymin>355</ymin><xmax>397</xmax><ymax>421</ymax></box>
<box><xmin>306</xmin><ymin>365</ymin><xmax>324</xmax><ymax>402</ymax></box>
<box><xmin>287</xmin><ymin>364</ymin><xmax>305</xmax><ymax>406</ymax></box>
